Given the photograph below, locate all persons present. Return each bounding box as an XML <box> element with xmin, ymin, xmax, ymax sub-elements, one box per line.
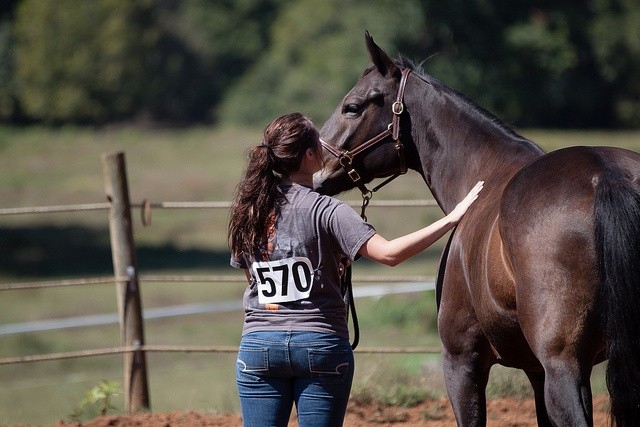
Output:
<box><xmin>227</xmin><ymin>112</ymin><xmax>484</xmax><ymax>427</ymax></box>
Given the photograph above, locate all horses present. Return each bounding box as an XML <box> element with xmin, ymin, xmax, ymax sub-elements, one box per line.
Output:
<box><xmin>312</xmin><ymin>30</ymin><xmax>640</xmax><ymax>427</ymax></box>
